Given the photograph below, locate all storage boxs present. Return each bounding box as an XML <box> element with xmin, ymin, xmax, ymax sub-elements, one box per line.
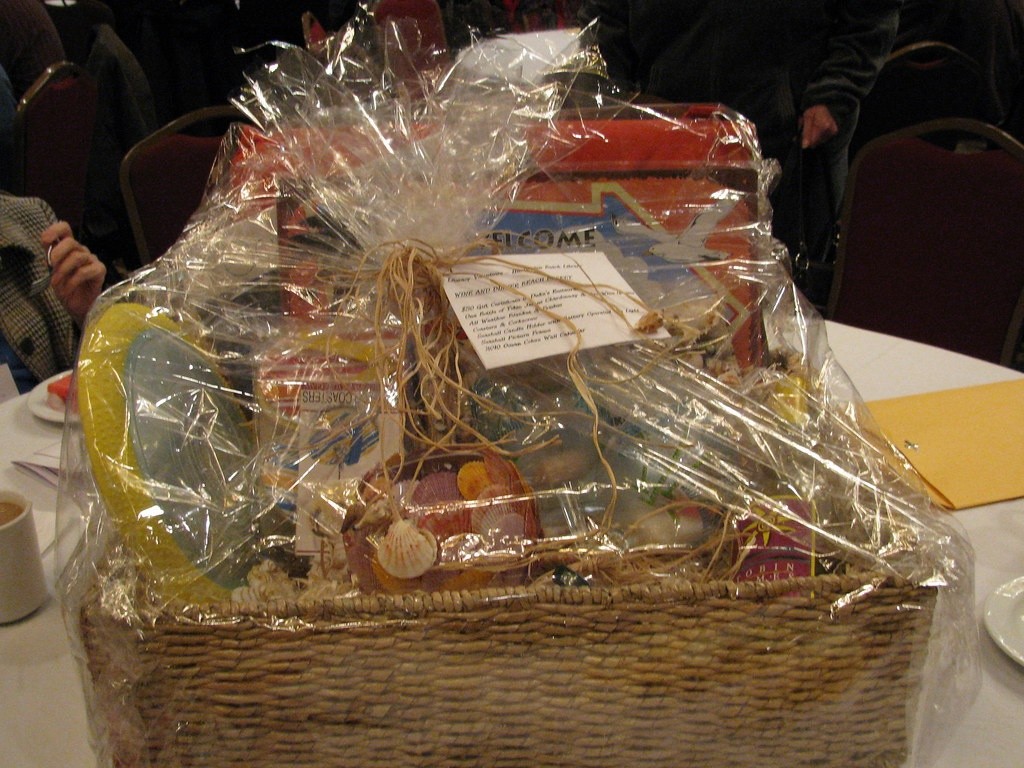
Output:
<box><xmin>232</xmin><ymin>112</ymin><xmax>770</xmax><ymax>366</ymax></box>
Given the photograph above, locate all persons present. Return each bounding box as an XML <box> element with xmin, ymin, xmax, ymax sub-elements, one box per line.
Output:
<box><xmin>0</xmin><ymin>190</ymin><xmax>107</xmax><ymax>395</ymax></box>
<box><xmin>896</xmin><ymin>0</ymin><xmax>1024</xmax><ymax>149</ymax></box>
<box><xmin>0</xmin><ymin>0</ymin><xmax>141</xmax><ymax>290</ymax></box>
<box><xmin>577</xmin><ymin>0</ymin><xmax>902</xmax><ymax>308</ymax></box>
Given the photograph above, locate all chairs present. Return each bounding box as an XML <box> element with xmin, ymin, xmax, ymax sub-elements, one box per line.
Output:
<box><xmin>119</xmin><ymin>108</ymin><xmax>265</xmax><ymax>268</ymax></box>
<box><xmin>848</xmin><ymin>40</ymin><xmax>1001</xmax><ymax>167</ymax></box>
<box><xmin>369</xmin><ymin>0</ymin><xmax>449</xmax><ymax>100</ymax></box>
<box><xmin>824</xmin><ymin>118</ymin><xmax>1023</xmax><ymax>369</ymax></box>
<box><xmin>15</xmin><ymin>62</ymin><xmax>99</xmax><ymax>245</ymax></box>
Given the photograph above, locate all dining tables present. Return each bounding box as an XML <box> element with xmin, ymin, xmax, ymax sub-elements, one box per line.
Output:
<box><xmin>0</xmin><ymin>314</ymin><xmax>1024</xmax><ymax>768</ymax></box>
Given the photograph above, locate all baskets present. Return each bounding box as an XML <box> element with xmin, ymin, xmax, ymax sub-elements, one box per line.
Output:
<box><xmin>78</xmin><ymin>528</ymin><xmax>937</xmax><ymax>768</ymax></box>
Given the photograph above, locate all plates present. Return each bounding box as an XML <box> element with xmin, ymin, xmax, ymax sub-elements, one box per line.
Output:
<box><xmin>122</xmin><ymin>328</ymin><xmax>256</xmax><ymax>591</ymax></box>
<box><xmin>983</xmin><ymin>576</ymin><xmax>1024</xmax><ymax>668</ymax></box>
<box><xmin>26</xmin><ymin>368</ymin><xmax>79</xmax><ymax>423</ymax></box>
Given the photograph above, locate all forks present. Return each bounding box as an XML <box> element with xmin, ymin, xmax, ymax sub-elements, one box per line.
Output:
<box><xmin>29</xmin><ymin>242</ymin><xmax>54</xmax><ymax>296</ymax></box>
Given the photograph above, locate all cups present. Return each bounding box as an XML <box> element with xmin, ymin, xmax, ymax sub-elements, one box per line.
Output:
<box><xmin>0</xmin><ymin>489</ymin><xmax>50</xmax><ymax>624</ymax></box>
